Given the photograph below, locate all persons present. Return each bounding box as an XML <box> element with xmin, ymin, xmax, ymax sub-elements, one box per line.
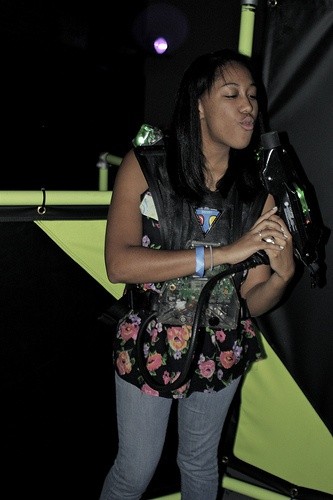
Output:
<box><xmin>98</xmin><ymin>49</ymin><xmax>294</xmax><ymax>500</ymax></box>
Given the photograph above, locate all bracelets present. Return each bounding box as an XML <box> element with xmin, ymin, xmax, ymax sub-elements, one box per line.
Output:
<box><xmin>205</xmin><ymin>245</ymin><xmax>213</xmax><ymax>272</ymax></box>
<box><xmin>195</xmin><ymin>246</ymin><xmax>205</xmax><ymax>277</ymax></box>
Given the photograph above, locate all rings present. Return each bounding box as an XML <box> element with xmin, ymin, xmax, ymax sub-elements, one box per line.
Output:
<box><xmin>259</xmin><ymin>231</ymin><xmax>263</xmax><ymax>239</ymax></box>
